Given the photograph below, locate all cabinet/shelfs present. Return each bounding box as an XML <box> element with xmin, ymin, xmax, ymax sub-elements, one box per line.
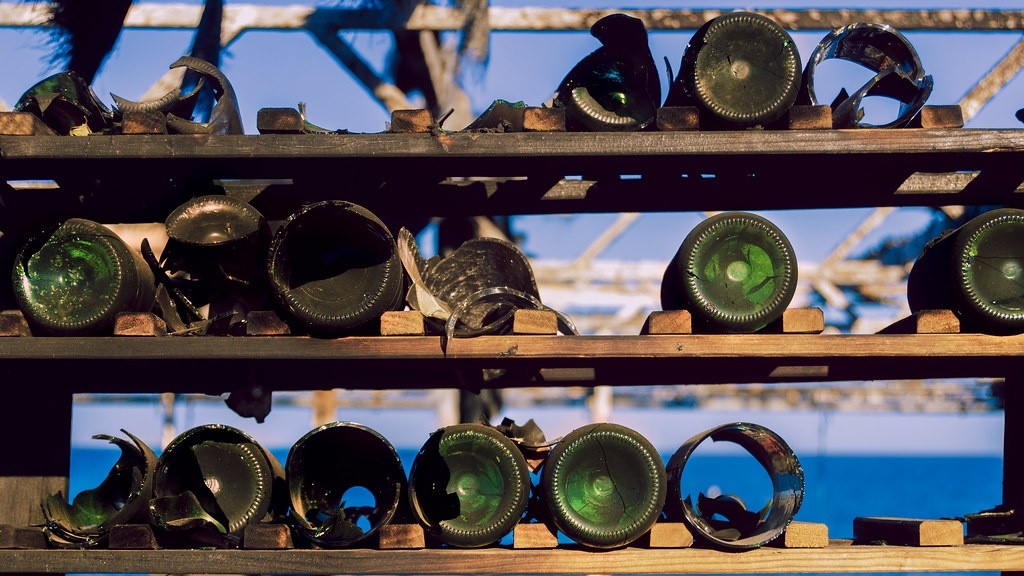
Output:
<box><xmin>0</xmin><ymin>103</ymin><xmax>1024</xmax><ymax>576</ymax></box>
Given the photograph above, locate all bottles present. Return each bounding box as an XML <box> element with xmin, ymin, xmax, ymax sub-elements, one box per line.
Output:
<box><xmin>268</xmin><ymin>201</ymin><xmax>404</xmax><ymax>331</ymax></box>
<box><xmin>10</xmin><ymin>217</ymin><xmax>169</xmax><ymax>338</ymax></box>
<box><xmin>660</xmin><ymin>210</ymin><xmax>799</xmax><ymax>333</ymax></box>
<box><xmin>45</xmin><ymin>424</ymin><xmax>806</xmax><ymax>550</ymax></box>
<box><xmin>681</xmin><ymin>13</ymin><xmax>804</xmax><ymax>128</ymax></box>
<box><xmin>907</xmin><ymin>206</ymin><xmax>1024</xmax><ymax>334</ymax></box>
